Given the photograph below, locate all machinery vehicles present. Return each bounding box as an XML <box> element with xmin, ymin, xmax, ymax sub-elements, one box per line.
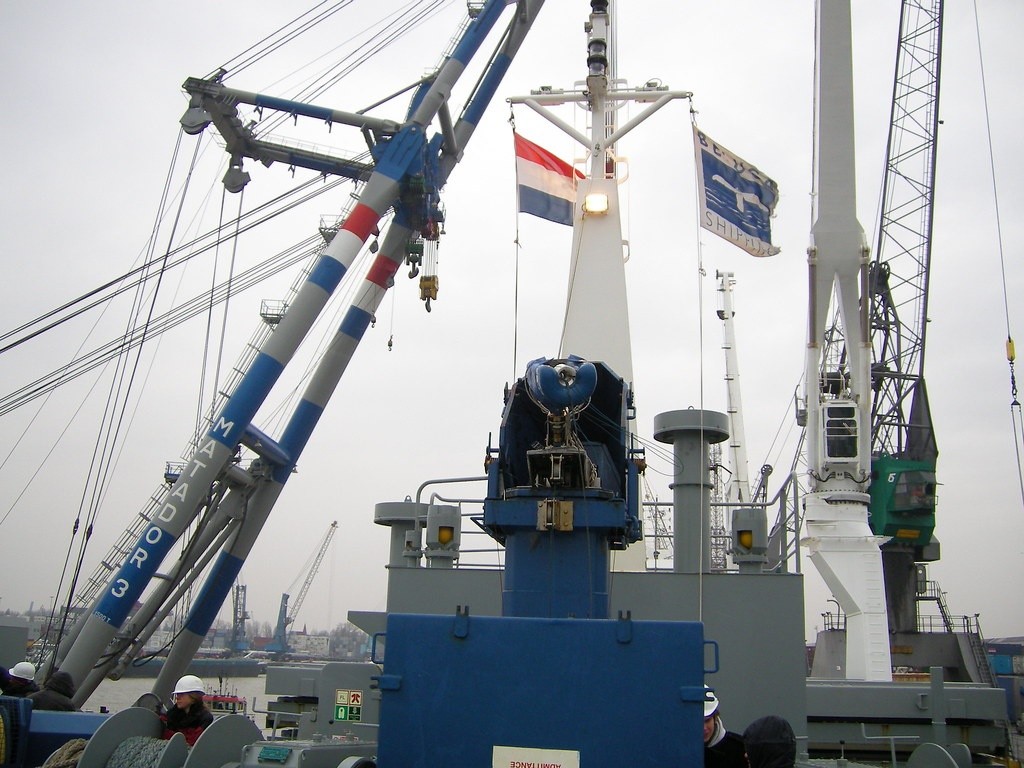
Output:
<box><xmin>227</xmin><ymin>573</ymin><xmax>253</xmax><ymax>653</ymax></box>
<box><xmin>263</xmin><ymin>519</ymin><xmax>340</xmax><ymax>660</ymax></box>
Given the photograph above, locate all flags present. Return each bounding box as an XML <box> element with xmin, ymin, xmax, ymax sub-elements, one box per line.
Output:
<box><xmin>693</xmin><ymin>126</ymin><xmax>781</xmax><ymax>257</ymax></box>
<box><xmin>514</xmin><ymin>132</ymin><xmax>585</xmax><ymax>226</ymax></box>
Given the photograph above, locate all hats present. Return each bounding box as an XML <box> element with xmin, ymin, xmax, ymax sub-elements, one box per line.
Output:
<box><xmin>44</xmin><ymin>670</ymin><xmax>75</xmax><ymax>697</ymax></box>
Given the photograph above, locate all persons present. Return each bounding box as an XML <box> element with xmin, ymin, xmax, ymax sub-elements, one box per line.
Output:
<box><xmin>160</xmin><ymin>675</ymin><xmax>213</xmax><ymax>746</ymax></box>
<box><xmin>704</xmin><ymin>683</ymin><xmax>796</xmax><ymax>768</ymax></box>
<box><xmin>0</xmin><ymin>662</ymin><xmax>75</xmax><ymax>711</ymax></box>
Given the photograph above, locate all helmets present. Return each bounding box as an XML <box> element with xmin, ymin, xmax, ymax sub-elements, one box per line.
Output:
<box><xmin>703</xmin><ymin>684</ymin><xmax>720</xmax><ymax>720</ymax></box>
<box><xmin>9</xmin><ymin>662</ymin><xmax>36</xmax><ymax>680</ymax></box>
<box><xmin>173</xmin><ymin>676</ymin><xmax>206</xmax><ymax>696</ymax></box>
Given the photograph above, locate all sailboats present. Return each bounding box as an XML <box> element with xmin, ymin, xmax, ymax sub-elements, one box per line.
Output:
<box><xmin>0</xmin><ymin>0</ymin><xmax>1023</xmax><ymax>768</ymax></box>
